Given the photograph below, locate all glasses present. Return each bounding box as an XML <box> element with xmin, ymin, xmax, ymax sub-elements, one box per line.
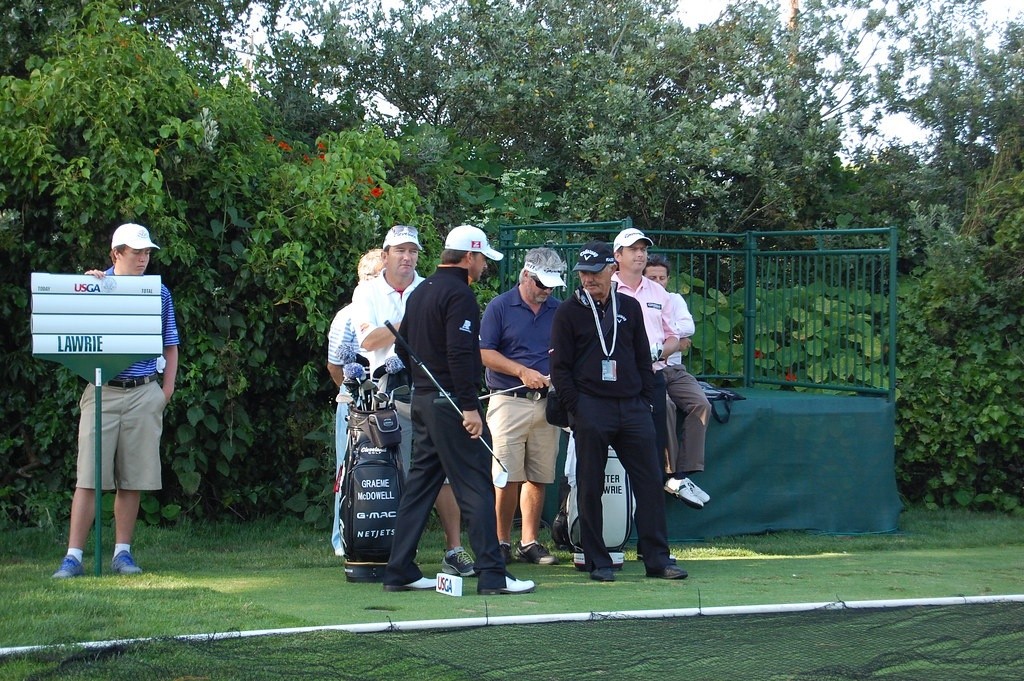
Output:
<box><xmin>531</xmin><ymin>275</ymin><xmax>554</xmax><ymax>290</ymax></box>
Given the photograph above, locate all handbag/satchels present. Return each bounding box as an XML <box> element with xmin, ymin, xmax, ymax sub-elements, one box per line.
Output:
<box><xmin>545</xmin><ymin>390</ymin><xmax>569</xmax><ymax>428</ymax></box>
<box><xmin>369</xmin><ymin>409</ymin><xmax>404</xmax><ymax>449</ymax></box>
<box><xmin>697</xmin><ymin>380</ymin><xmax>747</xmax><ymax>424</ymax></box>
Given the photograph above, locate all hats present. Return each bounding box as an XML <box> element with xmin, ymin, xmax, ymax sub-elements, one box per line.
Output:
<box><xmin>381</xmin><ymin>224</ymin><xmax>422</xmax><ymax>250</ymax></box>
<box><xmin>444</xmin><ymin>224</ymin><xmax>503</xmax><ymax>261</ymax></box>
<box><xmin>525</xmin><ymin>260</ymin><xmax>567</xmax><ymax>287</ymax></box>
<box><xmin>570</xmin><ymin>241</ymin><xmax>615</xmax><ymax>272</ymax></box>
<box><xmin>111</xmin><ymin>222</ymin><xmax>160</xmax><ymax>250</ymax></box>
<box><xmin>614</xmin><ymin>228</ymin><xmax>653</xmax><ymax>253</ymax></box>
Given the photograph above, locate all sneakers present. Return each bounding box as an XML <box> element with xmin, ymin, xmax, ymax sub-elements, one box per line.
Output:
<box><xmin>500</xmin><ymin>543</ymin><xmax>513</xmax><ymax>564</ymax></box>
<box><xmin>50</xmin><ymin>554</ymin><xmax>84</xmax><ymax>579</ymax></box>
<box><xmin>663</xmin><ymin>476</ymin><xmax>710</xmax><ymax>510</ymax></box>
<box><xmin>442</xmin><ymin>547</ymin><xmax>476</xmax><ymax>577</ymax></box>
<box><xmin>513</xmin><ymin>539</ymin><xmax>559</xmax><ymax>565</ymax></box>
<box><xmin>111</xmin><ymin>550</ymin><xmax>142</xmax><ymax>574</ymax></box>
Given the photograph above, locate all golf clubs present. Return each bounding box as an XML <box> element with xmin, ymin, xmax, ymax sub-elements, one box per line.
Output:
<box><xmin>479</xmin><ymin>377</ymin><xmax>551</xmax><ymax>402</ymax></box>
<box><xmin>383</xmin><ymin>317</ymin><xmax>510</xmax><ymax>490</ymax></box>
<box><xmin>357</xmin><ymin>378</ymin><xmax>411</xmax><ymax>413</ymax></box>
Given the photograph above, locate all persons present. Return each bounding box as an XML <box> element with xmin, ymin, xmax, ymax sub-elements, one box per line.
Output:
<box><xmin>550</xmin><ymin>240</ymin><xmax>688</xmax><ymax>582</ymax></box>
<box><xmin>382</xmin><ymin>224</ymin><xmax>536</xmax><ymax>595</ymax></box>
<box><xmin>642</xmin><ymin>255</ymin><xmax>711</xmax><ymax>510</ymax></box>
<box><xmin>352</xmin><ymin>226</ymin><xmax>475</xmax><ymax>576</ymax></box>
<box><xmin>328</xmin><ymin>249</ymin><xmax>385</xmax><ymax>555</ymax></box>
<box><xmin>611</xmin><ymin>228</ymin><xmax>680</xmax><ymax>565</ymax></box>
<box><xmin>479</xmin><ymin>248</ymin><xmax>567</xmax><ymax>564</ymax></box>
<box><xmin>52</xmin><ymin>223</ymin><xmax>180</xmax><ymax>578</ymax></box>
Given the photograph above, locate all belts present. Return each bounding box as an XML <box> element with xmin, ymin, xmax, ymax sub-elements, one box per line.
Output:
<box><xmin>108</xmin><ymin>374</ymin><xmax>156</xmax><ymax>388</ymax></box>
<box><xmin>492</xmin><ymin>389</ymin><xmax>547</xmax><ymax>400</ymax></box>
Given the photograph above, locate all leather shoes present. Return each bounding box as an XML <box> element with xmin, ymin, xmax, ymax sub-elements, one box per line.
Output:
<box><xmin>591</xmin><ymin>568</ymin><xmax>615</xmax><ymax>581</ymax></box>
<box><xmin>385</xmin><ymin>577</ymin><xmax>438</xmax><ymax>591</ymax></box>
<box><xmin>657</xmin><ymin>564</ymin><xmax>689</xmax><ymax>578</ymax></box>
<box><xmin>477</xmin><ymin>576</ymin><xmax>534</xmax><ymax>595</ymax></box>
